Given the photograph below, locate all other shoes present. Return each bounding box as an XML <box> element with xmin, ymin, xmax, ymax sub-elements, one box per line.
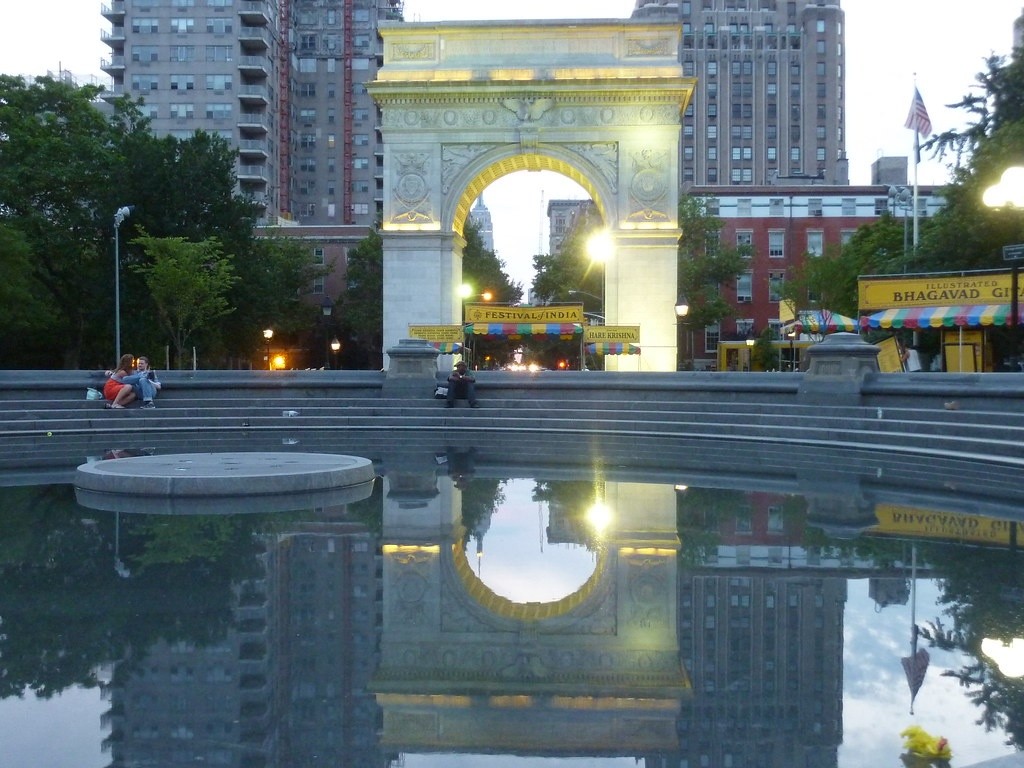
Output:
<box><xmin>471</xmin><ymin>403</ymin><xmax>479</xmax><ymax>408</ymax></box>
<box><xmin>104</xmin><ymin>402</ymin><xmax>127</xmax><ymax>409</ymax></box>
<box><xmin>444</xmin><ymin>404</ymin><xmax>454</xmax><ymax>408</ymax></box>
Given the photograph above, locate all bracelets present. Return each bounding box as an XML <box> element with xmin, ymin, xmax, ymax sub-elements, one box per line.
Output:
<box><xmin>459</xmin><ymin>375</ymin><xmax>462</xmax><ymax>379</ymax></box>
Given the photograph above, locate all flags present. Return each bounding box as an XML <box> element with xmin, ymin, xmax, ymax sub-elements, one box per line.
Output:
<box><xmin>904</xmin><ymin>86</ymin><xmax>932</xmax><ymax>140</ymax></box>
<box><xmin>901</xmin><ymin>647</ymin><xmax>929</xmax><ymax>699</ymax></box>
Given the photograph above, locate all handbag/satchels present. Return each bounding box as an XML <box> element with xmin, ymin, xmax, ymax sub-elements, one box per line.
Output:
<box><xmin>86</xmin><ymin>388</ymin><xmax>102</xmax><ymax>400</ymax></box>
<box><xmin>435</xmin><ymin>387</ymin><xmax>449</xmax><ymax>399</ymax></box>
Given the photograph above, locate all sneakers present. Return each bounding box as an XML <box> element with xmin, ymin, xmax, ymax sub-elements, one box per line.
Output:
<box><xmin>140</xmin><ymin>401</ymin><xmax>155</xmax><ymax>409</ymax></box>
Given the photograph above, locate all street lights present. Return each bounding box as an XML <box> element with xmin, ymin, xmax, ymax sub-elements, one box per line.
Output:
<box><xmin>263</xmin><ymin>327</ymin><xmax>274</xmax><ymax>371</ymax></box>
<box><xmin>787</xmin><ymin>332</ymin><xmax>796</xmax><ymax>372</ymax></box>
<box><xmin>746</xmin><ymin>333</ymin><xmax>756</xmax><ymax>372</ymax></box>
<box><xmin>982</xmin><ymin>165</ymin><xmax>1024</xmax><ymax>373</ymax></box>
<box><xmin>977</xmin><ymin>519</ymin><xmax>1024</xmax><ymax>680</ymax></box>
<box><xmin>331</xmin><ymin>334</ymin><xmax>341</xmax><ymax>370</ymax></box>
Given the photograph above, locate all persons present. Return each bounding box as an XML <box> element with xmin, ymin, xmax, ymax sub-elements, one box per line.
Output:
<box><xmin>103</xmin><ymin>353</ymin><xmax>136</xmax><ymax>409</ymax></box>
<box><xmin>446</xmin><ymin>361</ymin><xmax>482</xmax><ymax>409</ymax></box>
<box><xmin>900</xmin><ymin>345</ymin><xmax>923</xmax><ymax>372</ymax></box>
<box><xmin>122</xmin><ymin>357</ymin><xmax>162</xmax><ymax>408</ymax></box>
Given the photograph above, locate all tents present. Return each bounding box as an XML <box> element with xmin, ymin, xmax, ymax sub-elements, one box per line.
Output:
<box><xmin>584</xmin><ymin>342</ymin><xmax>642</xmax><ymax>357</ymax></box>
<box><xmin>427</xmin><ymin>342</ymin><xmax>473</xmax><ymax>356</ymax></box>
<box><xmin>465</xmin><ymin>321</ymin><xmax>584</xmax><ymax>341</ymax></box>
<box><xmin>780</xmin><ymin>307</ymin><xmax>862</xmax><ymax>373</ymax></box>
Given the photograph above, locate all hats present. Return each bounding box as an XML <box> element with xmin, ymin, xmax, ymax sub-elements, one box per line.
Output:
<box><xmin>454</xmin><ymin>361</ymin><xmax>466</xmax><ymax>367</ymax></box>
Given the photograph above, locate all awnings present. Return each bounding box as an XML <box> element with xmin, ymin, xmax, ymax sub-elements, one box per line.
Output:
<box><xmin>862</xmin><ymin>303</ymin><xmax>1024</xmax><ymax>329</ymax></box>
